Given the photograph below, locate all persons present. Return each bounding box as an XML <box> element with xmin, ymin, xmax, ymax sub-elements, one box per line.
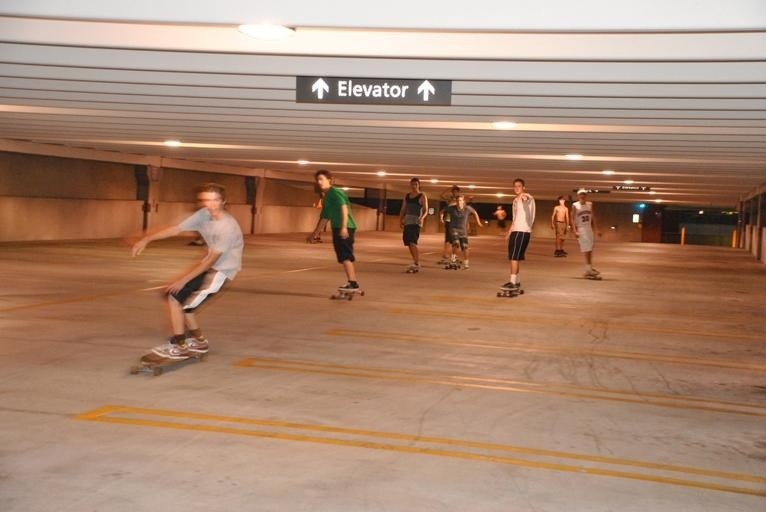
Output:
<box><xmin>499</xmin><ymin>179</ymin><xmax>536</xmax><ymax>291</ymax></box>
<box><xmin>131</xmin><ymin>184</ymin><xmax>245</xmax><ymax>361</ymax></box>
<box><xmin>440</xmin><ymin>186</ymin><xmax>466</xmax><ymax>259</ymax></box>
<box><xmin>399</xmin><ymin>178</ymin><xmax>429</xmax><ymax>270</ymax></box>
<box><xmin>571</xmin><ymin>188</ymin><xmax>599</xmax><ymax>276</ymax></box>
<box><xmin>439</xmin><ymin>195</ymin><xmax>483</xmax><ymax>269</ymax></box>
<box><xmin>313</xmin><ymin>199</ymin><xmax>326</xmax><ymax>240</ymax></box>
<box><xmin>306</xmin><ymin>170</ymin><xmax>360</xmax><ymax>292</ymax></box>
<box><xmin>551</xmin><ymin>197</ymin><xmax>569</xmax><ymax>256</ymax></box>
<box><xmin>492</xmin><ymin>205</ymin><xmax>507</xmax><ymax>236</ymax></box>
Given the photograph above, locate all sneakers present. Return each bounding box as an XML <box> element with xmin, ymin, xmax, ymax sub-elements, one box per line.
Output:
<box><xmin>555</xmin><ymin>250</ymin><xmax>567</xmax><ymax>255</ymax></box>
<box><xmin>585</xmin><ymin>269</ymin><xmax>600</xmax><ymax>275</ymax></box>
<box><xmin>500</xmin><ymin>282</ymin><xmax>520</xmax><ymax>290</ymax></box>
<box><xmin>151</xmin><ymin>338</ymin><xmax>209</xmax><ymax>359</ymax></box>
<box><xmin>338</xmin><ymin>281</ymin><xmax>359</xmax><ymax>291</ymax></box>
<box><xmin>408</xmin><ymin>264</ymin><xmax>420</xmax><ymax>270</ymax></box>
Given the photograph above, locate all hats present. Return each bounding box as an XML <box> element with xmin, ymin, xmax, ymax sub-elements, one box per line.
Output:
<box><xmin>577</xmin><ymin>189</ymin><xmax>587</xmax><ymax>194</ymax></box>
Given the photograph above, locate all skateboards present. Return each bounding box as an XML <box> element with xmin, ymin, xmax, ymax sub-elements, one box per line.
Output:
<box><xmin>495</xmin><ymin>285</ymin><xmax>523</xmax><ymax>297</ymax></box>
<box><xmin>401</xmin><ymin>264</ymin><xmax>421</xmax><ymax>274</ymax></box>
<box><xmin>554</xmin><ymin>251</ymin><xmax>566</xmax><ymax>257</ymax></box>
<box><xmin>131</xmin><ymin>338</ymin><xmax>208</xmax><ymax>376</ymax></box>
<box><xmin>445</xmin><ymin>258</ymin><xmax>462</xmax><ymax>270</ymax></box>
<box><xmin>583</xmin><ymin>270</ymin><xmax>602</xmax><ymax>280</ymax></box>
<box><xmin>331</xmin><ymin>285</ymin><xmax>364</xmax><ymax>301</ymax></box>
<box><xmin>438</xmin><ymin>257</ymin><xmax>450</xmax><ymax>264</ymax></box>
